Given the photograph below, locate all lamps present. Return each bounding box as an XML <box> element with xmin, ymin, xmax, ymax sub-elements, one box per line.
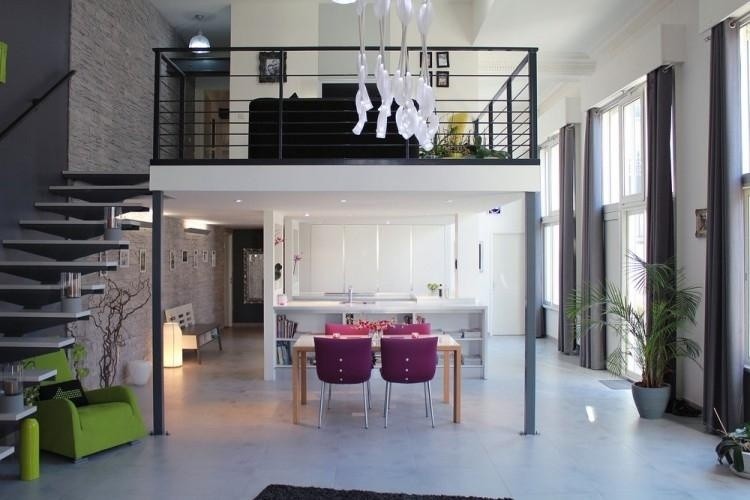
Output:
<box><xmin>347</xmin><ymin>0</ymin><xmax>441</xmax><ymax>154</ymax></box>
<box><xmin>188</xmin><ymin>12</ymin><xmax>210</xmax><ymax>55</ymax></box>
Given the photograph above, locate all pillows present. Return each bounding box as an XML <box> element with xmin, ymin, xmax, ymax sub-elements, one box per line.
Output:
<box><xmin>37</xmin><ymin>378</ymin><xmax>90</xmax><ymax>409</ymax></box>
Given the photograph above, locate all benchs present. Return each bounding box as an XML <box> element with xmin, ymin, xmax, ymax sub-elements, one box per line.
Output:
<box><xmin>245</xmin><ymin>93</ymin><xmax>420</xmax><ymax>160</ymax></box>
<box><xmin>163</xmin><ymin>300</ymin><xmax>223</xmax><ymax>364</ymax></box>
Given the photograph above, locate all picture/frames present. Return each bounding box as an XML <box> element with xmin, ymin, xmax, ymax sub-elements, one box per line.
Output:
<box><xmin>419</xmin><ymin>50</ymin><xmax>452</xmax><ymax>89</ymax></box>
<box><xmin>168</xmin><ymin>248</ymin><xmax>219</xmax><ymax>271</ymax></box>
<box><xmin>97</xmin><ymin>240</ymin><xmax>147</xmax><ymax>275</ymax></box>
<box><xmin>256</xmin><ymin>50</ymin><xmax>286</xmax><ymax>84</ymax></box>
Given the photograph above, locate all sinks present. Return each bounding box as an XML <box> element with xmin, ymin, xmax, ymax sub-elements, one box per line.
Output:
<box><xmin>339</xmin><ymin>300</ymin><xmax>375</xmax><ymax>304</ymax></box>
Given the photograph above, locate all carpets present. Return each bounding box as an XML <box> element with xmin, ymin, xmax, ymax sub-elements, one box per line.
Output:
<box><xmin>596</xmin><ymin>378</ymin><xmax>633</xmax><ymax>392</ymax></box>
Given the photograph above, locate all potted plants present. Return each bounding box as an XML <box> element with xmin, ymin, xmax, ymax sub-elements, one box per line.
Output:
<box><xmin>718</xmin><ymin>425</ymin><xmax>749</xmax><ymax>479</ymax></box>
<box><xmin>562</xmin><ymin>245</ymin><xmax>706</xmax><ymax>420</ymax></box>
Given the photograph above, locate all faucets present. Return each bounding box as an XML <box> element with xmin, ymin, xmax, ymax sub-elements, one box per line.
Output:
<box><xmin>347</xmin><ymin>285</ymin><xmax>353</xmax><ymax>301</ymax></box>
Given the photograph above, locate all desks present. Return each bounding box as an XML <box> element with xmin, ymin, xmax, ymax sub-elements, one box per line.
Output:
<box><xmin>288</xmin><ymin>331</ymin><xmax>461</xmax><ymax>427</ymax></box>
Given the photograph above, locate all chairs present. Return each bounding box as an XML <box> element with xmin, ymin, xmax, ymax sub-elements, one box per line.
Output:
<box><xmin>312</xmin><ymin>320</ymin><xmax>438</xmax><ymax>434</ymax></box>
<box><xmin>19</xmin><ymin>345</ymin><xmax>153</xmax><ymax>465</ymax></box>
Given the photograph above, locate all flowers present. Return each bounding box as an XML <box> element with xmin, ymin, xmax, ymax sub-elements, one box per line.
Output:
<box><xmin>419</xmin><ymin>111</ymin><xmax>512</xmax><ymax>161</ymax></box>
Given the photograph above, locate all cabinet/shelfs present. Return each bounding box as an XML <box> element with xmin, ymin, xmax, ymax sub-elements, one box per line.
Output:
<box><xmin>271</xmin><ymin>305</ymin><xmax>490</xmax><ymax>382</ymax></box>
<box><xmin>381</xmin><ymin>223</ymin><xmax>447</xmax><ymax>293</ymax></box>
<box><xmin>307</xmin><ymin>223</ymin><xmax>379</xmax><ymax>293</ymax></box>
